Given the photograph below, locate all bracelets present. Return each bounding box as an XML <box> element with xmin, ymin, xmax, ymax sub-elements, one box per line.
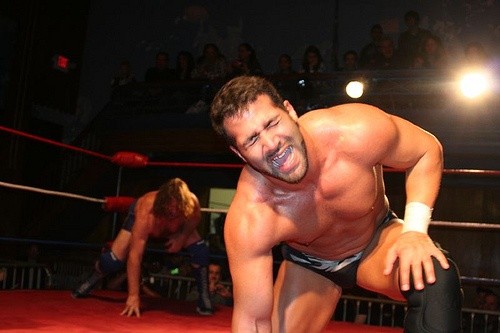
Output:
<box><xmin>400</xmin><ymin>201</ymin><xmax>433</xmax><ymax>234</ymax></box>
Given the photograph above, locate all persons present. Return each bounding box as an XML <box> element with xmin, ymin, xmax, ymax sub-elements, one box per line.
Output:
<box><xmin>0</xmin><ymin>205</ymin><xmax>500</xmax><ymax>333</ymax></box>
<box><xmin>104</xmin><ymin>5</ymin><xmax>500</xmax><ymax>127</ymax></box>
<box><xmin>70</xmin><ymin>177</ymin><xmax>213</xmax><ymax>319</ymax></box>
<box><xmin>211</xmin><ymin>75</ymin><xmax>466</xmax><ymax>332</ymax></box>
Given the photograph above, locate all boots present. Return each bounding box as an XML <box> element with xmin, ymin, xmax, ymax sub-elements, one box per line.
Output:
<box><xmin>193</xmin><ymin>266</ymin><xmax>212</xmax><ymax>315</ymax></box>
<box><xmin>72</xmin><ymin>260</ymin><xmax>106</xmax><ymax>298</ymax></box>
